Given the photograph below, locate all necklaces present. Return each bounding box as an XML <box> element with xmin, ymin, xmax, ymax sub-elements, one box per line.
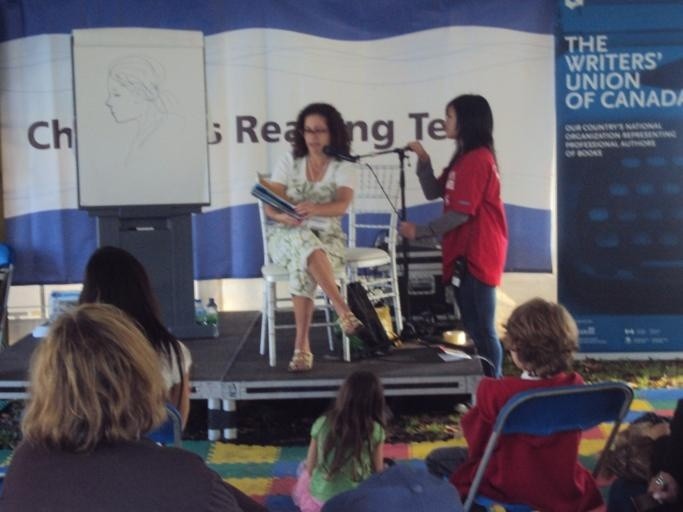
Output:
<box><xmin>305</xmin><ymin>156</ymin><xmax>330</xmax><ymax>190</ymax></box>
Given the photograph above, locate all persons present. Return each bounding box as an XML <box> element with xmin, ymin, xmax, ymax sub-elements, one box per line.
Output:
<box><xmin>1</xmin><ymin>303</ymin><xmax>243</xmax><ymax>510</ymax></box>
<box><xmin>81</xmin><ymin>245</ymin><xmax>192</xmax><ymax>432</ymax></box>
<box><xmin>292</xmin><ymin>371</ymin><xmax>388</xmax><ymax>512</ymax></box>
<box><xmin>398</xmin><ymin>96</ymin><xmax>508</xmax><ymax>415</ymax></box>
<box><xmin>424</xmin><ymin>298</ymin><xmax>602</xmax><ymax>512</ymax></box>
<box><xmin>104</xmin><ymin>57</ymin><xmax>185</xmax><ymax>155</ymax></box>
<box><xmin>263</xmin><ymin>104</ymin><xmax>365</xmax><ymax>372</ymax></box>
<box><xmin>608</xmin><ymin>396</ymin><xmax>683</xmax><ymax>512</ymax></box>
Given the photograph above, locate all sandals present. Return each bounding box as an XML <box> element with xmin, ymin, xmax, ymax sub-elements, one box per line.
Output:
<box><xmin>339</xmin><ymin>314</ymin><xmax>366</xmax><ymax>337</ymax></box>
<box><xmin>289</xmin><ymin>349</ymin><xmax>314</xmax><ymax>372</ymax></box>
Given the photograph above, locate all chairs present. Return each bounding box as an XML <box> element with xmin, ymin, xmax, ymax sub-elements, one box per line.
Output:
<box><xmin>456</xmin><ymin>381</ymin><xmax>635</xmax><ymax>512</ymax></box>
<box><xmin>251</xmin><ymin>170</ymin><xmax>351</xmax><ymax>368</ymax></box>
<box><xmin>329</xmin><ymin>160</ymin><xmax>411</xmax><ymax>346</ymax></box>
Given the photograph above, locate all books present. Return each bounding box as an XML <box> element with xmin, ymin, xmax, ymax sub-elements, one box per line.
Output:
<box><xmin>251</xmin><ymin>183</ymin><xmax>307</xmax><ymax>222</ymax></box>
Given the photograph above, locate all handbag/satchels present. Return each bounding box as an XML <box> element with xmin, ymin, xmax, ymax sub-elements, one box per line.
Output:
<box><xmin>608</xmin><ymin>412</ymin><xmax>672</xmax><ymax>479</ymax></box>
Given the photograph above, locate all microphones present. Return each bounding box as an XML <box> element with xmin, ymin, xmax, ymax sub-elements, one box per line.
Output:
<box><xmin>324</xmin><ymin>145</ymin><xmax>359</xmax><ymax>164</ymax></box>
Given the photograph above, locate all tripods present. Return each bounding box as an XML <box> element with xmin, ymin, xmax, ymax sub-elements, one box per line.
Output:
<box><xmin>352</xmin><ymin>152</ymin><xmax>473</xmax><ymax>359</ymax></box>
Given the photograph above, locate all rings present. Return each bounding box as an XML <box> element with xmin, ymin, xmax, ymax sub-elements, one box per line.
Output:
<box><xmin>655</xmin><ymin>476</ymin><xmax>664</xmax><ymax>487</ymax></box>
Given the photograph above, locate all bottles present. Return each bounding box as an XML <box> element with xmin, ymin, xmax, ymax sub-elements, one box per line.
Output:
<box><xmin>205</xmin><ymin>296</ymin><xmax>219</xmax><ymax>339</ymax></box>
<box><xmin>195</xmin><ymin>299</ymin><xmax>205</xmax><ymax>324</ymax></box>
